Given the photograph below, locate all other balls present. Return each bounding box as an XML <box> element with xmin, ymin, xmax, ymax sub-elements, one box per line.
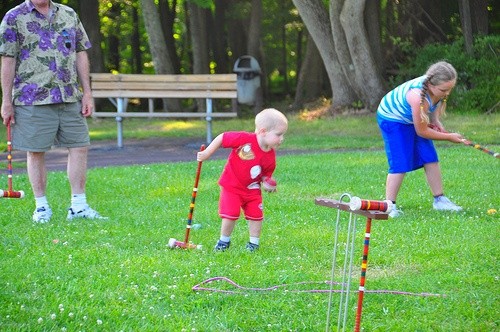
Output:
<box><xmin>487</xmin><ymin>208</ymin><xmax>497</xmax><ymax>215</ymax></box>
<box><xmin>262</xmin><ymin>178</ymin><xmax>277</xmax><ymax>193</ymax></box>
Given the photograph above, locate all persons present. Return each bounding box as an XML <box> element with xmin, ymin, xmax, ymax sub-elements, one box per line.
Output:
<box><xmin>197</xmin><ymin>108</ymin><xmax>288</xmax><ymax>252</ymax></box>
<box><xmin>0</xmin><ymin>0</ymin><xmax>109</xmax><ymax>225</ymax></box>
<box><xmin>375</xmin><ymin>61</ymin><xmax>466</xmax><ymax>216</ymax></box>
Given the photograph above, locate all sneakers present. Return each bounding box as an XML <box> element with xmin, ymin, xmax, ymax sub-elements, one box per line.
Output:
<box><xmin>33</xmin><ymin>206</ymin><xmax>52</xmax><ymax>223</ymax></box>
<box><xmin>389</xmin><ymin>204</ymin><xmax>404</xmax><ymax>218</ymax></box>
<box><xmin>66</xmin><ymin>204</ymin><xmax>109</xmax><ymax>221</ymax></box>
<box><xmin>433</xmin><ymin>196</ymin><xmax>463</xmax><ymax>211</ymax></box>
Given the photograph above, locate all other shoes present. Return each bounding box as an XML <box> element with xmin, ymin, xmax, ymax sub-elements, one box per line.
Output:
<box><xmin>214</xmin><ymin>240</ymin><xmax>231</xmax><ymax>252</ymax></box>
<box><xmin>246</xmin><ymin>240</ymin><xmax>259</xmax><ymax>250</ymax></box>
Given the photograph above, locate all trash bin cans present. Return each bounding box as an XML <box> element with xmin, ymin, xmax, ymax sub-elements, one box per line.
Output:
<box><xmin>233</xmin><ymin>55</ymin><xmax>262</xmax><ymax>104</ymax></box>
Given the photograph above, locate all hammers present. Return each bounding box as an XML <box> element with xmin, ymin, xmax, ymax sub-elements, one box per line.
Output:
<box><xmin>0</xmin><ymin>120</ymin><xmax>25</xmax><ymax>198</ymax></box>
<box><xmin>429</xmin><ymin>124</ymin><xmax>500</xmax><ymax>158</ymax></box>
<box><xmin>349</xmin><ymin>197</ymin><xmax>392</xmax><ymax>332</ymax></box>
<box><xmin>169</xmin><ymin>144</ymin><xmax>205</xmax><ymax>250</ymax></box>
